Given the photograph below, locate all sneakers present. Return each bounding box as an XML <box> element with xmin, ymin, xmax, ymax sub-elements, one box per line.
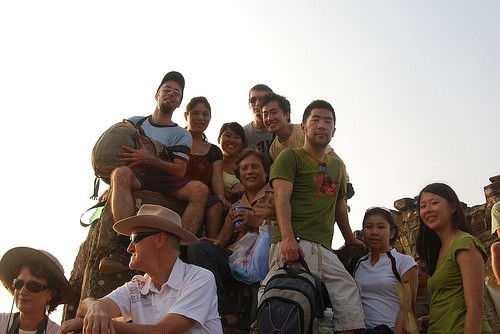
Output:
<box><xmin>99</xmin><ymin>251</ymin><xmax>130</xmax><ymax>274</ymax></box>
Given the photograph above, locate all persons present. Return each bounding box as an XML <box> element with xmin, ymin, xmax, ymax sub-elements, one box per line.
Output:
<box><xmin>0</xmin><ymin>246</ymin><xmax>75</xmax><ymax>334</ymax></box>
<box><xmin>99</xmin><ymin>71</ymin><xmax>209</xmax><ymax>275</ymax></box>
<box><xmin>241</xmin><ymin>84</ymin><xmax>275</xmax><ymax>168</ymax></box>
<box><xmin>490</xmin><ymin>200</ymin><xmax>500</xmax><ymax>285</ymax></box>
<box><xmin>187</xmin><ymin>147</ymin><xmax>277</xmax><ymax>334</ymax></box>
<box><xmin>57</xmin><ymin>204</ymin><xmax>223</xmax><ymax>334</ymax></box>
<box><xmin>260</xmin><ymin>92</ymin><xmax>355</xmax><ymax>200</ymax></box>
<box><xmin>170</xmin><ymin>96</ymin><xmax>232</xmax><ymax>241</ymax></box>
<box><xmin>346</xmin><ymin>207</ymin><xmax>419</xmax><ymax>334</ymax></box>
<box><xmin>415</xmin><ymin>183</ymin><xmax>494</xmax><ymax>334</ymax></box>
<box><xmin>218</xmin><ymin>121</ymin><xmax>248</xmax><ymax>226</ymax></box>
<box><xmin>257</xmin><ymin>99</ymin><xmax>368</xmax><ymax>334</ymax></box>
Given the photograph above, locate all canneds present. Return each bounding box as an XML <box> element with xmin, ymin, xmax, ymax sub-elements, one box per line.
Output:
<box><xmin>233</xmin><ymin>206</ymin><xmax>244</xmax><ymax>226</ymax></box>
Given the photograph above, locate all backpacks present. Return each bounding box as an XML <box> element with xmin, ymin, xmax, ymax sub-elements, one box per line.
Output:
<box><xmin>89</xmin><ymin>115</ymin><xmax>191</xmax><ymax>200</ymax></box>
<box><xmin>257</xmin><ymin>255</ymin><xmax>335</xmax><ymax>334</ymax></box>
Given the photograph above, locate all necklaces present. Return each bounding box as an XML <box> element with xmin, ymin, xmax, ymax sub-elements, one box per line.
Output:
<box><xmin>190</xmin><ymin>141</ymin><xmax>206</xmax><ymax>155</ymax></box>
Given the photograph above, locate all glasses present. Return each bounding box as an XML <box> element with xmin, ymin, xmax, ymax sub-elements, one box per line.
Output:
<box><xmin>249</xmin><ymin>97</ymin><xmax>265</xmax><ymax>103</ymax></box>
<box><xmin>12</xmin><ymin>279</ymin><xmax>48</xmax><ymax>292</ymax></box>
<box><xmin>130</xmin><ymin>231</ymin><xmax>160</xmax><ymax>244</ymax></box>
<box><xmin>319</xmin><ymin>163</ymin><xmax>332</xmax><ymax>188</ymax></box>
<box><xmin>160</xmin><ymin>87</ymin><xmax>182</xmax><ymax>97</ymax></box>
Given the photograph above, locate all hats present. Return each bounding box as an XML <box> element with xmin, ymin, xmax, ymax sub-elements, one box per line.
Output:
<box><xmin>157</xmin><ymin>71</ymin><xmax>185</xmax><ymax>91</ymax></box>
<box><xmin>113</xmin><ymin>204</ymin><xmax>199</xmax><ymax>246</ymax></box>
<box><xmin>0</xmin><ymin>247</ymin><xmax>75</xmax><ymax>304</ymax></box>
